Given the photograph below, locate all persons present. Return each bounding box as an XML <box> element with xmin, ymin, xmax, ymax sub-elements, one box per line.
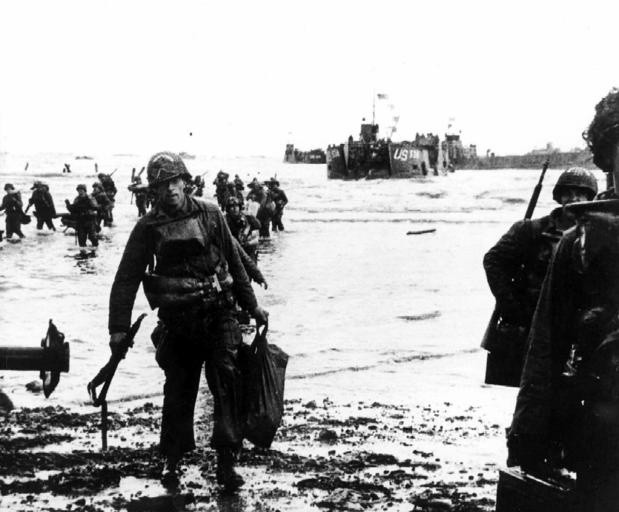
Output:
<box><xmin>58</xmin><ymin>169</ymin><xmax>289</xmax><ymax>257</ymax></box>
<box><xmin>503</xmin><ymin>84</ymin><xmax>618</xmax><ymax>512</ymax></box>
<box><xmin>0</xmin><ymin>183</ymin><xmax>25</xmax><ymax>240</ymax></box>
<box><xmin>232</xmin><ymin>235</ymin><xmax>268</xmax><ymax>326</ymax></box>
<box><xmin>105</xmin><ymin>151</ymin><xmax>274</xmax><ymax>492</ymax></box>
<box><xmin>29</xmin><ymin>180</ymin><xmax>58</xmax><ymax>232</ymax></box>
<box><xmin>221</xmin><ymin>194</ymin><xmax>264</xmax><ymax>282</ymax></box>
<box><xmin>476</xmin><ymin>165</ymin><xmax>596</xmax><ymax>388</ymax></box>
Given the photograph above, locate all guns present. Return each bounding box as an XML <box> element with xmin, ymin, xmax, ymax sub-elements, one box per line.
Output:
<box><xmin>88</xmin><ymin>313</ymin><xmax>147</xmax><ymax>407</ymax></box>
<box><xmin>481</xmin><ymin>159</ymin><xmax>550</xmax><ymax>352</ymax></box>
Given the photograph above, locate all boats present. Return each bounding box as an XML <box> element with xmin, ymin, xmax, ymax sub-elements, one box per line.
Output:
<box><xmin>324</xmin><ymin>93</ymin><xmax>461</xmax><ymax>185</ymax></box>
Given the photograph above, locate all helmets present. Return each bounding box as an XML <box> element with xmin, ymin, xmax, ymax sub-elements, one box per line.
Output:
<box><xmin>147</xmin><ymin>151</ymin><xmax>190</xmax><ymax>188</ymax></box>
<box><xmin>581</xmin><ymin>90</ymin><xmax>617</xmax><ymax>171</ymax></box>
<box><xmin>550</xmin><ymin>166</ymin><xmax>598</xmax><ymax>202</ymax></box>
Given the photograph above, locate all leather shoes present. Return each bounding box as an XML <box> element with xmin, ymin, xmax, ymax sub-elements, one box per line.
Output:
<box><xmin>216</xmin><ymin>457</ymin><xmax>245</xmax><ymax>491</ymax></box>
